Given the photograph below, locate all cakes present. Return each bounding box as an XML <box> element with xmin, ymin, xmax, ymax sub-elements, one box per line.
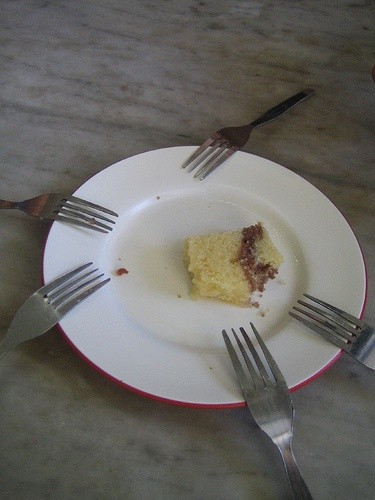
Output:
<box><xmin>186</xmin><ymin>221</ymin><xmax>283</xmax><ymax>307</ymax></box>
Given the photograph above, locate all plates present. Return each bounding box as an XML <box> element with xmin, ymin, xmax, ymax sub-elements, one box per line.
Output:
<box><xmin>41</xmin><ymin>145</ymin><xmax>368</xmax><ymax>410</ymax></box>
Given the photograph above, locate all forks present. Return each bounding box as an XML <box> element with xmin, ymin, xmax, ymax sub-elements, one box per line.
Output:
<box><xmin>1</xmin><ymin>192</ymin><xmax>118</xmax><ymax>234</ymax></box>
<box><xmin>288</xmin><ymin>292</ymin><xmax>374</xmax><ymax>374</ymax></box>
<box><xmin>182</xmin><ymin>86</ymin><xmax>317</xmax><ymax>181</ymax></box>
<box><xmin>221</xmin><ymin>321</ymin><xmax>311</xmax><ymax>499</ymax></box>
<box><xmin>1</xmin><ymin>261</ymin><xmax>111</xmax><ymax>359</ymax></box>
